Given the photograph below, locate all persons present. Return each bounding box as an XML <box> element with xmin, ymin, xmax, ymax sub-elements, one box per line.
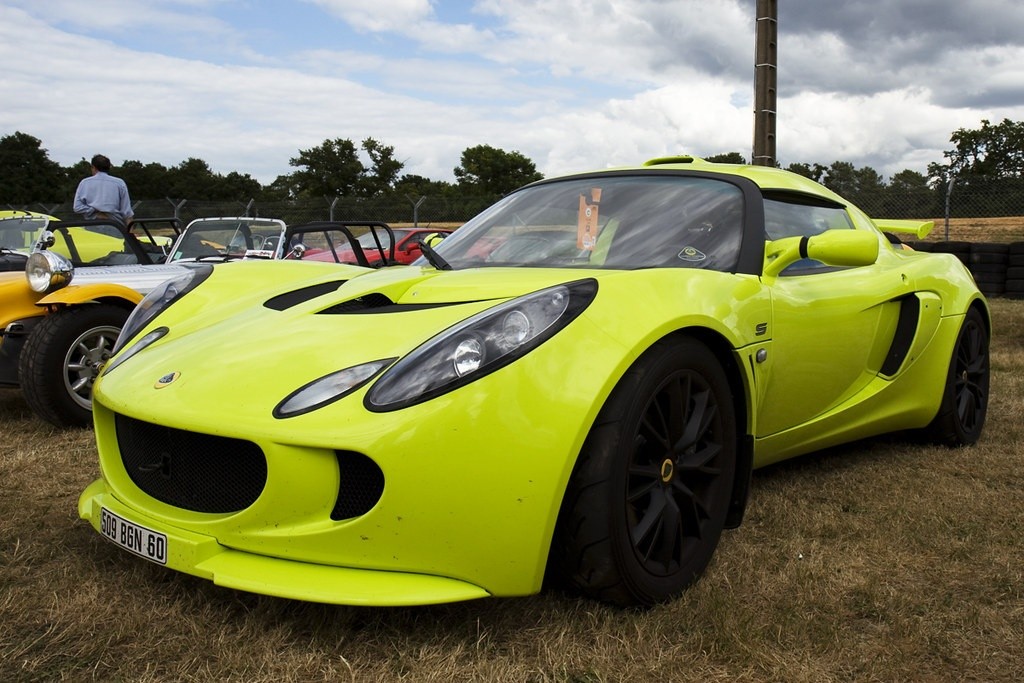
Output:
<box><xmin>73</xmin><ymin>154</ymin><xmax>135</xmax><ymax>239</ymax></box>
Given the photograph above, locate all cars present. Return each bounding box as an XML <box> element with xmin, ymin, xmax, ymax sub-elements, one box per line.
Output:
<box><xmin>0</xmin><ymin>209</ymin><xmax>172</xmax><ymax>269</ymax></box>
<box><xmin>298</xmin><ymin>224</ymin><xmax>503</xmax><ymax>275</ymax></box>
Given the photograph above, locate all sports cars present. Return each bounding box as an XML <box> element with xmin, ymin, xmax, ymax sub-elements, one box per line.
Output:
<box><xmin>77</xmin><ymin>152</ymin><xmax>990</xmax><ymax>606</ymax></box>
<box><xmin>1</xmin><ymin>215</ymin><xmax>306</xmax><ymax>437</ymax></box>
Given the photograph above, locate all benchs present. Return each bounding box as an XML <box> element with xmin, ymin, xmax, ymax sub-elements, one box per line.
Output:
<box><xmin>102</xmin><ymin>249</ymin><xmax>165</xmax><ymax>264</ymax></box>
